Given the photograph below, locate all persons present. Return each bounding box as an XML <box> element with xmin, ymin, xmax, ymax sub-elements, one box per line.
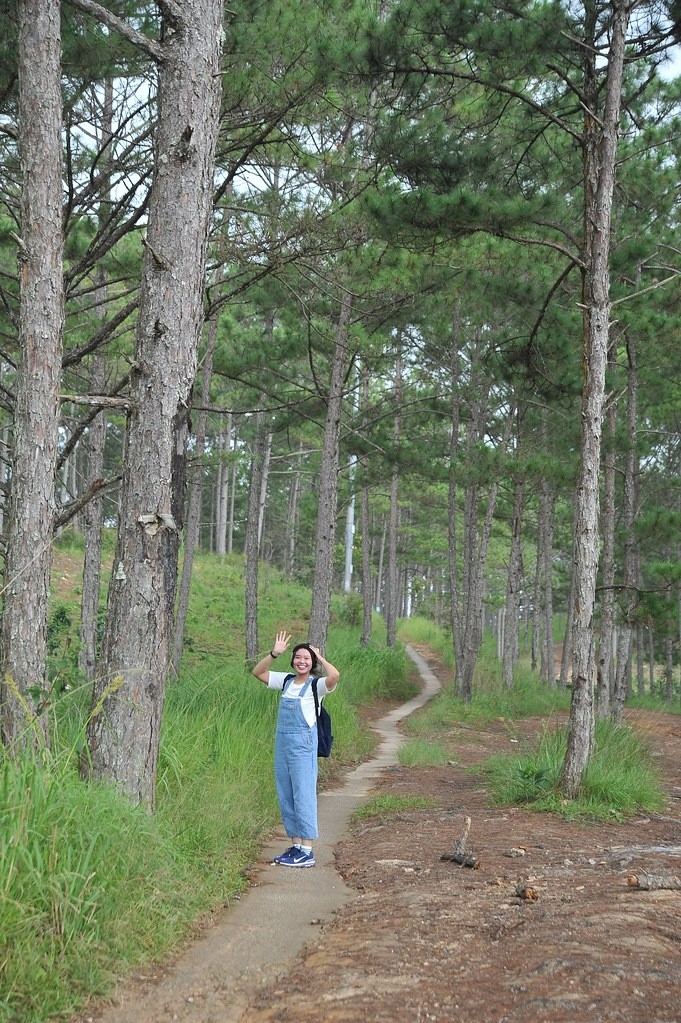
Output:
<box><xmin>252</xmin><ymin>630</ymin><xmax>340</xmax><ymax>868</ymax></box>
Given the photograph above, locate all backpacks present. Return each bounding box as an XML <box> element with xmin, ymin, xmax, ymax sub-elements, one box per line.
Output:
<box><xmin>283</xmin><ymin>672</ymin><xmax>335</xmax><ymax>758</ymax></box>
<box><xmin>282</xmin><ymin>674</ymin><xmax>334</xmax><ymax>758</ymax></box>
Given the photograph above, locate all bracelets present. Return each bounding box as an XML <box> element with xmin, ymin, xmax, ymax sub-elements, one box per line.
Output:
<box><xmin>270</xmin><ymin>650</ymin><xmax>277</xmax><ymax>659</ymax></box>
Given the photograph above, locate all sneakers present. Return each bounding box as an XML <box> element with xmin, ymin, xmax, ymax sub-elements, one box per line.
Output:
<box><xmin>278</xmin><ymin>851</ymin><xmax>315</xmax><ymax>868</ymax></box>
<box><xmin>273</xmin><ymin>846</ymin><xmax>302</xmax><ymax>864</ymax></box>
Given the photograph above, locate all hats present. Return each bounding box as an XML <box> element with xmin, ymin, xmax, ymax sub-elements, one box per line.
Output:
<box><xmin>292</xmin><ymin>643</ymin><xmax>318</xmax><ymax>669</ymax></box>
